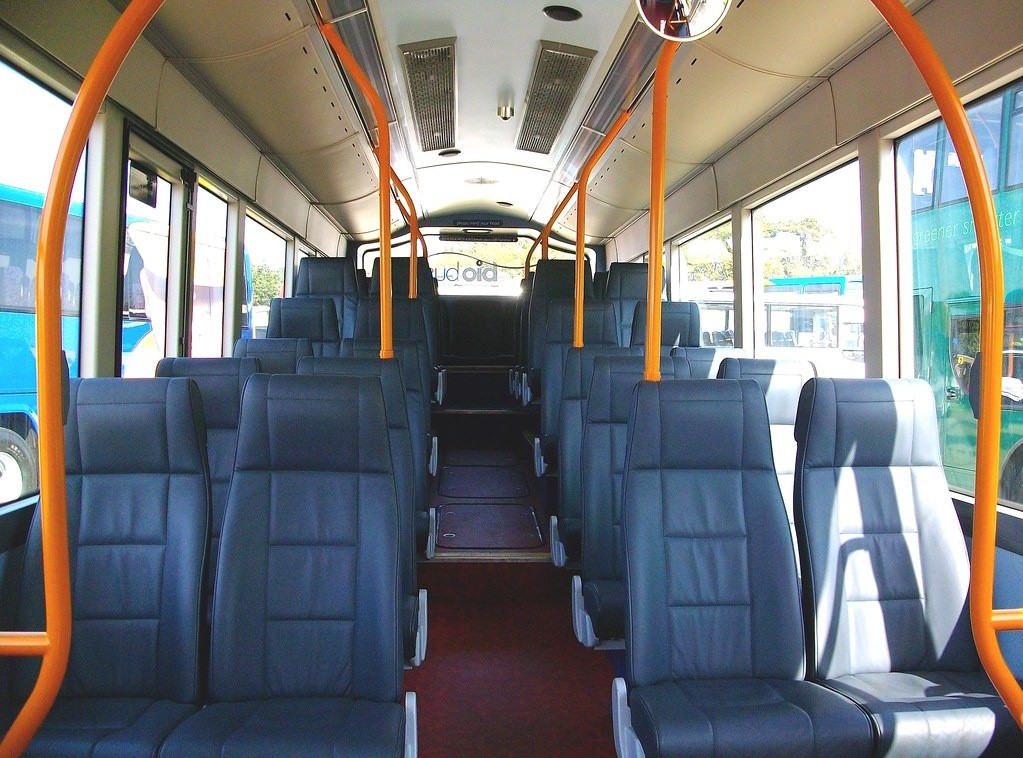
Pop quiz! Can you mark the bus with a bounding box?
[895,79,1022,514]
[0,182,254,505]
[682,293,865,426]
[683,274,862,334]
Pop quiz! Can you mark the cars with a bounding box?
[242,305,270,328]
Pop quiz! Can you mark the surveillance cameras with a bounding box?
[497,106,514,120]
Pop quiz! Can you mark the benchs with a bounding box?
[0,255,445,758]
[443,259,1023,757]
